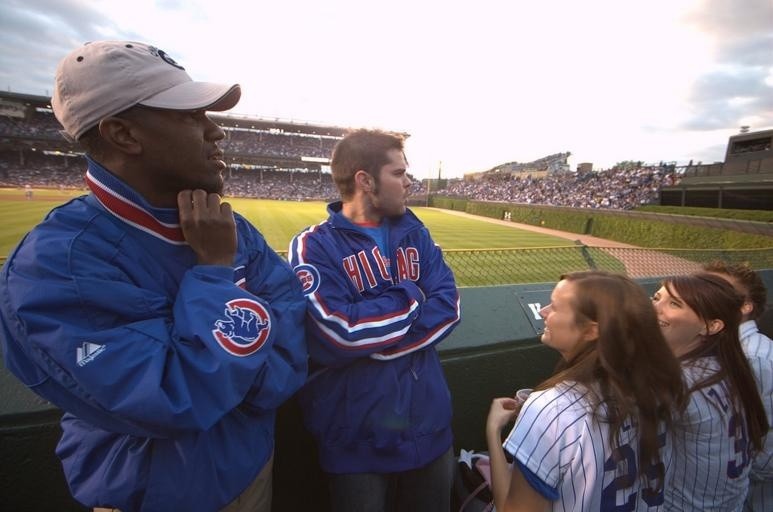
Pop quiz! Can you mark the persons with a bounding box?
[464,266,694,509]
[1,37,311,509]
[404,164,682,212]
[285,125,464,509]
[216,130,343,200]
[632,272,773,511]
[1,110,88,199]
[697,255,773,509]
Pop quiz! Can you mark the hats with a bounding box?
[49,40,241,144]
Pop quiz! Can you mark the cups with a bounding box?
[514,388,535,417]
